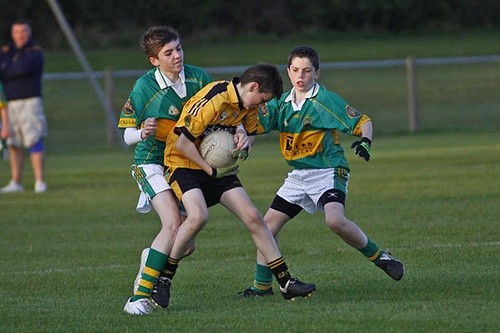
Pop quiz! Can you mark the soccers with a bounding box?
[199,131,238,168]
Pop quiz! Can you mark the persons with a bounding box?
[150,65,317,309]
[243,47,404,296]
[118,28,216,315]
[0,22,47,194]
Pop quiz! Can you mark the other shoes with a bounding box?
[34,182,46,193]
[0,183,24,192]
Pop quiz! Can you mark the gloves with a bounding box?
[231,148,251,161]
[350,137,372,162]
[211,165,239,178]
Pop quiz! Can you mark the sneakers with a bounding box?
[280,278,315,303]
[238,288,274,296]
[124,298,155,315]
[134,248,150,296]
[151,277,171,309]
[378,251,404,281]
